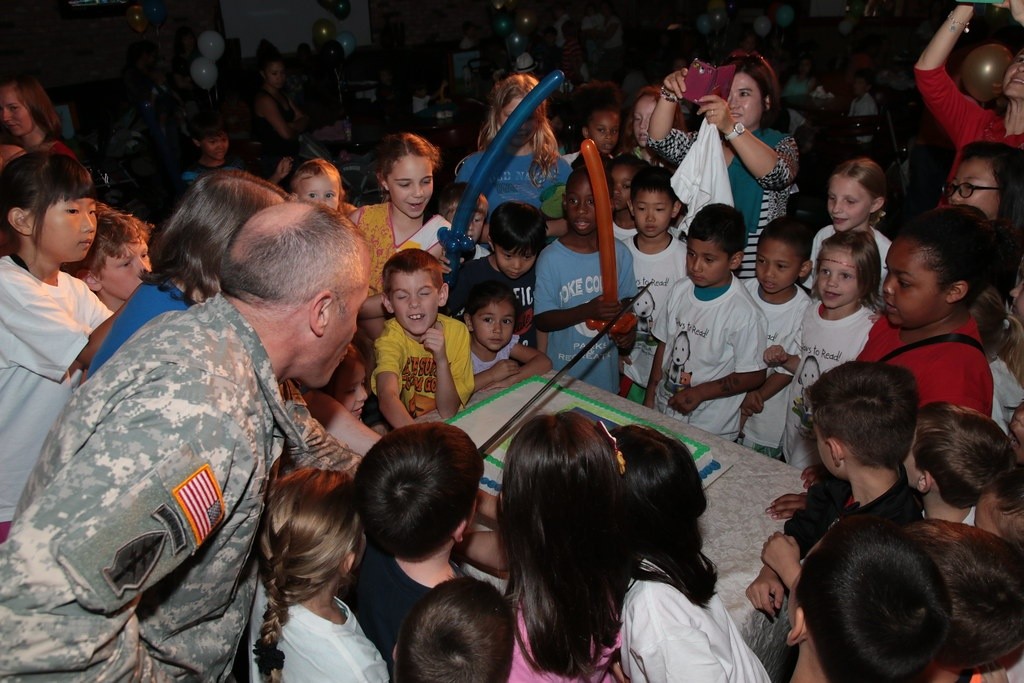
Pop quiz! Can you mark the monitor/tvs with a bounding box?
[58,0,137,21]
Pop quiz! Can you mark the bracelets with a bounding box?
[725,122,745,141]
[660,84,677,102]
[948,10,970,33]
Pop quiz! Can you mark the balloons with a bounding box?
[984,3,1009,29]
[726,1,737,15]
[697,14,711,34]
[197,30,225,61]
[768,1,784,24]
[190,57,218,90]
[320,0,336,10]
[336,30,356,56]
[127,4,148,32]
[505,0,518,9]
[319,40,344,68]
[707,0,726,15]
[508,32,529,56]
[331,0,350,20]
[438,69,565,283]
[581,139,636,336]
[515,7,538,35]
[711,8,728,31]
[313,18,336,46]
[775,5,795,28]
[961,43,1014,102]
[144,0,167,26]
[492,13,514,39]
[754,16,771,38]
[492,0,504,10]
[852,0,864,16]
[838,21,853,35]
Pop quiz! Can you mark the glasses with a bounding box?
[723,52,765,68]
[943,182,999,198]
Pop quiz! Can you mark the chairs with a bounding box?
[812,112,918,161]
[406,123,476,166]
[230,139,262,177]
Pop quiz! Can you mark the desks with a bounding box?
[356,367,812,683]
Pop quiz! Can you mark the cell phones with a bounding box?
[682,59,716,106]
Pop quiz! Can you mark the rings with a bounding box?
[711,110,713,115]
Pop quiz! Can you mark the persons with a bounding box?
[0,0,1024,683]
